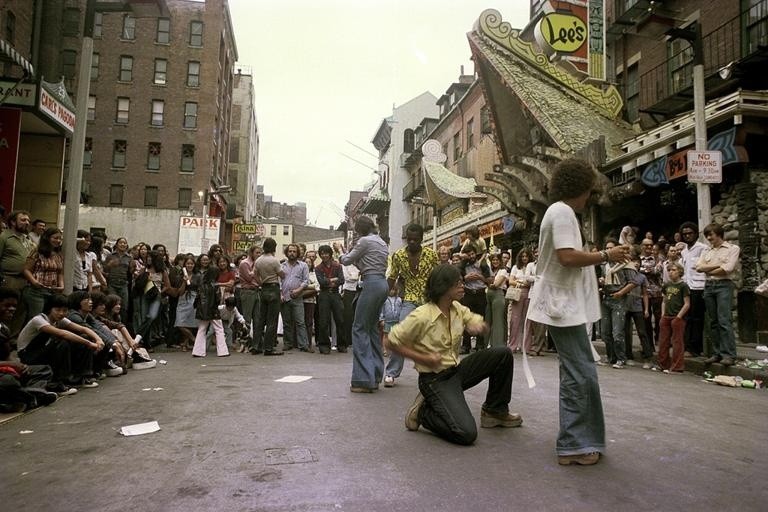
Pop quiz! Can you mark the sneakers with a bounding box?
[301,347,348,353]
[252,350,262,355]
[459,347,469,353]
[704,355,718,363]
[597,357,682,375]
[264,349,283,355]
[282,347,291,351]
[720,357,735,364]
[39,360,127,406]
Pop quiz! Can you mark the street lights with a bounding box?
[201,185,234,256]
[409,195,437,252]
[623,13,712,249]
[62,0,171,298]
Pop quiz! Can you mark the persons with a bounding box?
[520,157,633,465]
[382,264,525,444]
[2,211,740,414]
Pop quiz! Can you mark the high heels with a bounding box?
[559,453,599,465]
[384,375,394,386]
[133,354,156,371]
[350,385,377,394]
[480,408,522,429]
[405,390,425,431]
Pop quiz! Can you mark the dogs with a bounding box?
[237,327,253,354]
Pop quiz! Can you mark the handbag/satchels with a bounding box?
[504,287,522,301]
